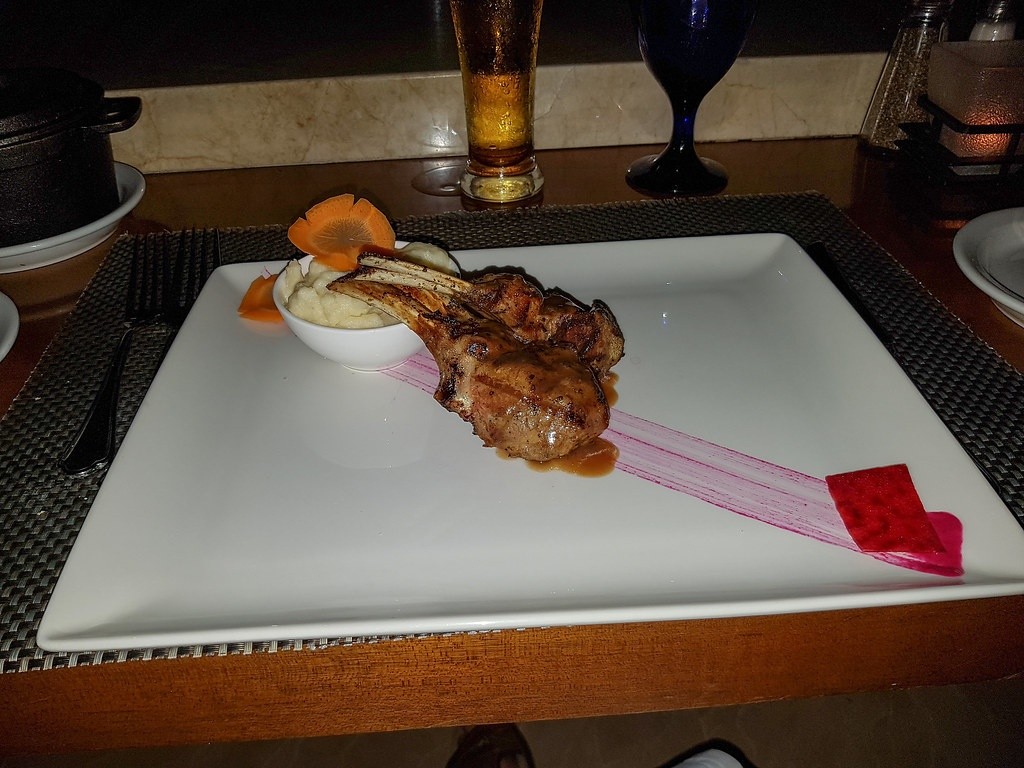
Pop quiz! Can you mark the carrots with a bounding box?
[288,193,396,273]
[238,274,287,322]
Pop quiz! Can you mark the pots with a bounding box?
[0,82,143,247]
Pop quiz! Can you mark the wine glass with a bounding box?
[625,0,758,196]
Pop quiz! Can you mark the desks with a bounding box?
[0,129,1024,768]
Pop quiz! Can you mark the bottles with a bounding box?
[969,1,1019,43]
[859,0,953,150]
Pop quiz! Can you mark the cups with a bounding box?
[927,41,1024,229]
[448,1,545,203]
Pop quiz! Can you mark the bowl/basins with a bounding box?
[272,241,461,371]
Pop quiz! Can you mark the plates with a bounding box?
[0,290,21,360]
[954,206,1024,328]
[1,160,145,273]
[34,232,1024,654]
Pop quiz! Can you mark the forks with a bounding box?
[58,234,171,477]
[154,227,222,374]
